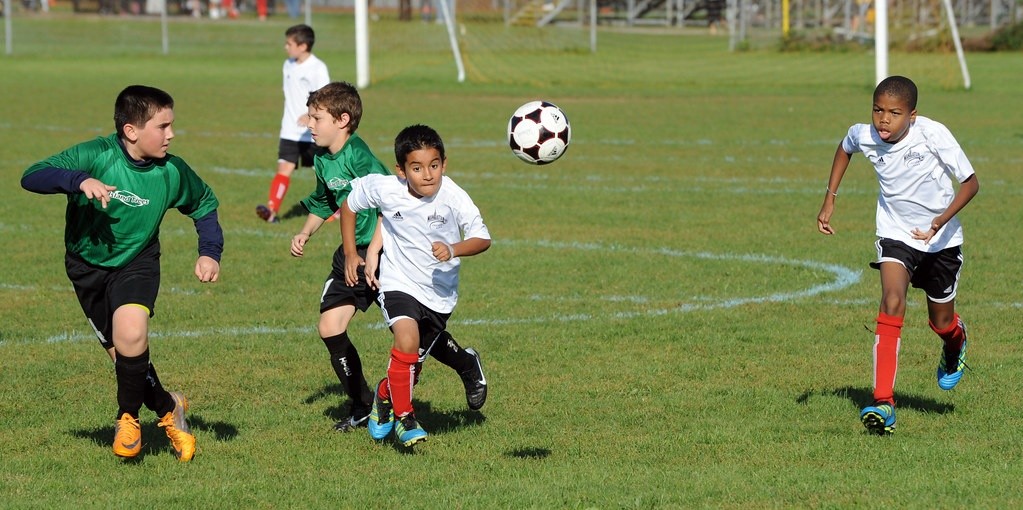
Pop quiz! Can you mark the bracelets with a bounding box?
[826,187,839,196]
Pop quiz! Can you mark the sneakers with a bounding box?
[368,377,394,438]
[393,411,427,447]
[157,391,195,461]
[255,205,279,222]
[938,320,967,390]
[458,347,487,410]
[112,412,142,456]
[333,411,372,433]
[860,404,896,435]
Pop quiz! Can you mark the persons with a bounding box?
[340,123,492,447]
[699,0,728,33]
[289,82,489,431]
[96,0,268,22]
[817,77,979,436]
[256,25,339,224]
[20,85,224,463]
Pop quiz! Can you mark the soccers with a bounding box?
[506,100,572,166]
